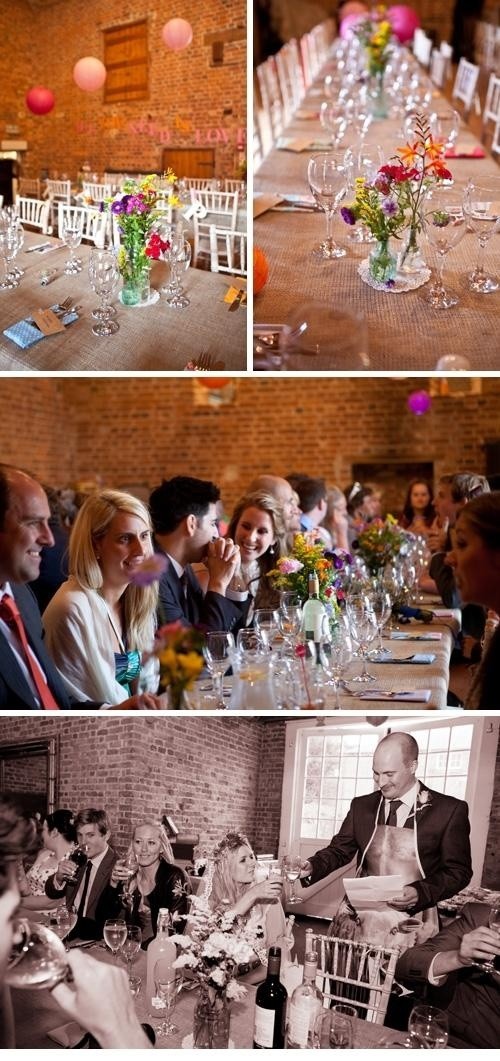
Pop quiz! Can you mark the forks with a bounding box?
[193,351,214,371]
[53,294,73,315]
[253,322,309,350]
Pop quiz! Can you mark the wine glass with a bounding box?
[118,853,139,900]
[283,855,305,906]
[316,31,463,190]
[160,232,192,308]
[90,244,122,338]
[1,205,26,293]
[306,153,351,261]
[200,532,430,708]
[61,212,85,276]
[343,142,385,244]
[63,842,91,881]
[2,905,143,1009]
[152,957,184,1037]
[458,174,500,292]
[419,191,466,310]
[472,897,499,975]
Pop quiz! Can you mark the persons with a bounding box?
[0,803,160,1050]
[179,831,296,991]
[46,807,123,939]
[93,819,192,953]
[290,732,475,1031]
[15,810,79,917]
[0,467,499,711]
[393,903,499,1049]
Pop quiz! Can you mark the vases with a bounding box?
[161,691,188,711]
[366,241,397,287]
[399,228,427,273]
[117,255,160,305]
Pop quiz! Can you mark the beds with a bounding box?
[0,223,249,370]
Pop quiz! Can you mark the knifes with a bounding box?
[26,244,52,254]
[59,306,82,319]
[228,289,244,313]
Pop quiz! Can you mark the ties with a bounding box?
[181,574,190,601]
[77,861,92,919]
[1,592,60,710]
[386,800,403,826]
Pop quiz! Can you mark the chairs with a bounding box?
[414,22,500,169]
[187,876,203,924]
[250,8,342,183]
[0,162,247,277]
[302,929,401,1030]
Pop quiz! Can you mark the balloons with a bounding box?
[407,390,432,415]
[25,86,57,116]
[73,56,109,93]
[337,1,420,44]
[162,17,194,52]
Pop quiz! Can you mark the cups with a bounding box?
[330,1004,358,1048]
[409,1005,451,1048]
[312,1011,353,1047]
[264,871,282,904]
[279,301,370,370]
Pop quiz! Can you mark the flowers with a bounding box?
[383,111,452,270]
[271,523,338,609]
[336,3,430,59]
[144,619,203,709]
[339,169,407,280]
[352,511,410,567]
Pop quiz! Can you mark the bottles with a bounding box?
[395,226,427,277]
[146,907,176,1019]
[287,949,323,1048]
[253,945,287,1048]
[367,243,399,285]
